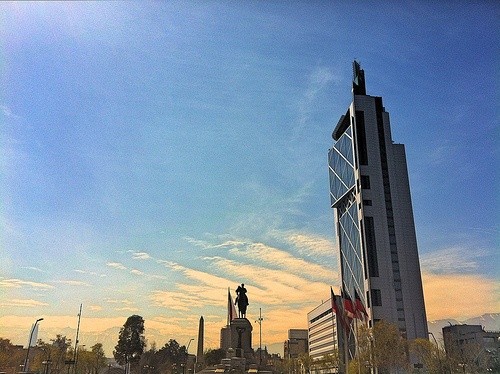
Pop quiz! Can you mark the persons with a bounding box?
[234,284,250,306]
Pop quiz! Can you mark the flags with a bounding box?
[342,282,363,322]
[330,288,347,333]
[339,288,353,341]
[352,287,369,324]
